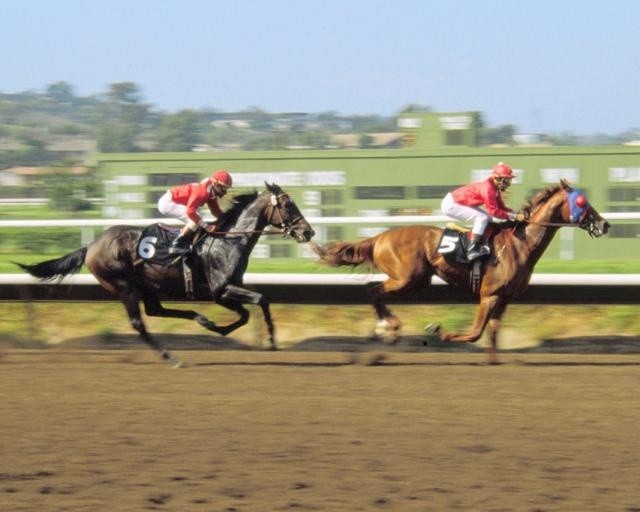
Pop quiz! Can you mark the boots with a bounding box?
[168,227,195,254]
[464,233,492,261]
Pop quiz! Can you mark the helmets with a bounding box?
[491,161,517,180]
[211,170,233,188]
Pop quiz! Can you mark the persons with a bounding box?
[439,161,527,262]
[155,170,233,254]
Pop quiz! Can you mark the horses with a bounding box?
[9,179,316,369]
[308,177,612,365]
